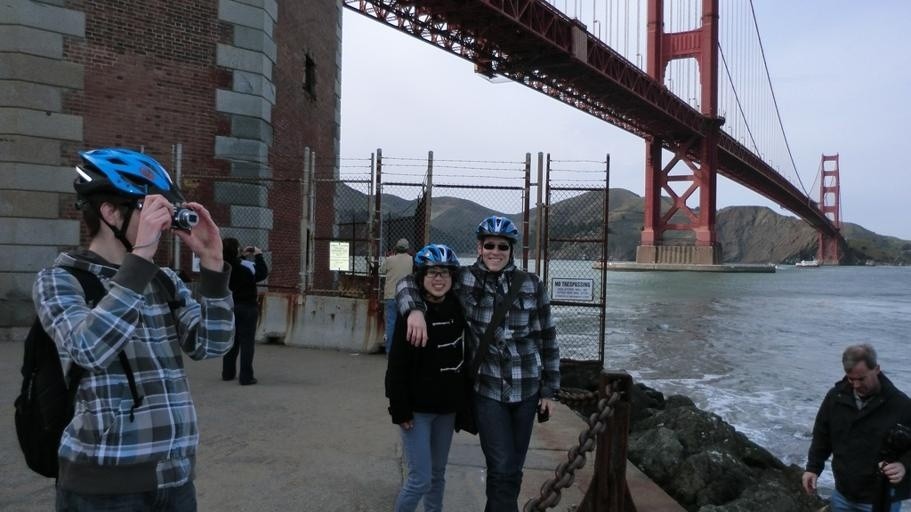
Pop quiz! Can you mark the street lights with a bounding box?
[668,79,675,92]
[693,98,698,109]
[727,125,793,183]
[593,20,601,41]
[636,53,643,70]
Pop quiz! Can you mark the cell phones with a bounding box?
[538,405,549,423]
[247,247,255,252]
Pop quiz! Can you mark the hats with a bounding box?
[396,238,410,251]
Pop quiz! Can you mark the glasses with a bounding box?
[425,271,453,278]
[484,243,511,251]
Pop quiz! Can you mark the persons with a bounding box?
[396,216,563,512]
[220,238,271,385]
[803,342,910,512]
[387,242,470,512]
[32,144,238,511]
[381,238,415,354]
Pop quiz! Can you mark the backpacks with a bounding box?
[13,264,177,480]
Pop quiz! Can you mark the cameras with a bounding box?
[170,201,199,231]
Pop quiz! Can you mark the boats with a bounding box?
[796,260,819,268]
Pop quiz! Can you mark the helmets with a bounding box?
[475,216,520,244]
[72,147,188,211]
[416,243,461,270]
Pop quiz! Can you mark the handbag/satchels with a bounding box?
[454,389,480,436]
